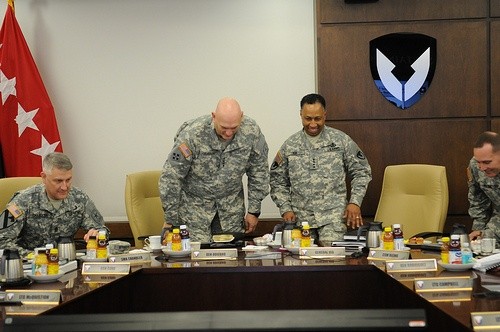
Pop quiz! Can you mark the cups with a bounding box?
[275,232,281,243]
[481,229,497,255]
[144,236,161,249]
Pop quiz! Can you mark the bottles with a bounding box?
[86,232,107,258]
[292,221,311,247]
[31,244,60,275]
[165,225,189,250]
[382,224,404,249]
[439,234,473,264]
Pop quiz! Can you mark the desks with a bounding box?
[0,247,500,332]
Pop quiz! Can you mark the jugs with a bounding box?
[0,247,24,282]
[273,221,296,245]
[366,221,384,247]
[53,233,76,261]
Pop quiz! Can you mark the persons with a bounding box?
[158,98,271,234]
[270,94,372,243]
[465,132,500,251]
[0,152,111,261]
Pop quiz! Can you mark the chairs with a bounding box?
[375,163,450,242]
[0,177,43,213]
[125,169,164,248]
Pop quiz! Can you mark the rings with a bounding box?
[356,217,359,219]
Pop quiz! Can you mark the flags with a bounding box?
[0,5,67,177]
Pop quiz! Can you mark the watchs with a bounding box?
[248,212,260,217]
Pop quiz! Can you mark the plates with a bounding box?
[27,271,64,283]
[435,260,476,273]
[252,237,318,253]
[376,246,412,251]
[404,241,423,246]
[79,245,191,258]
